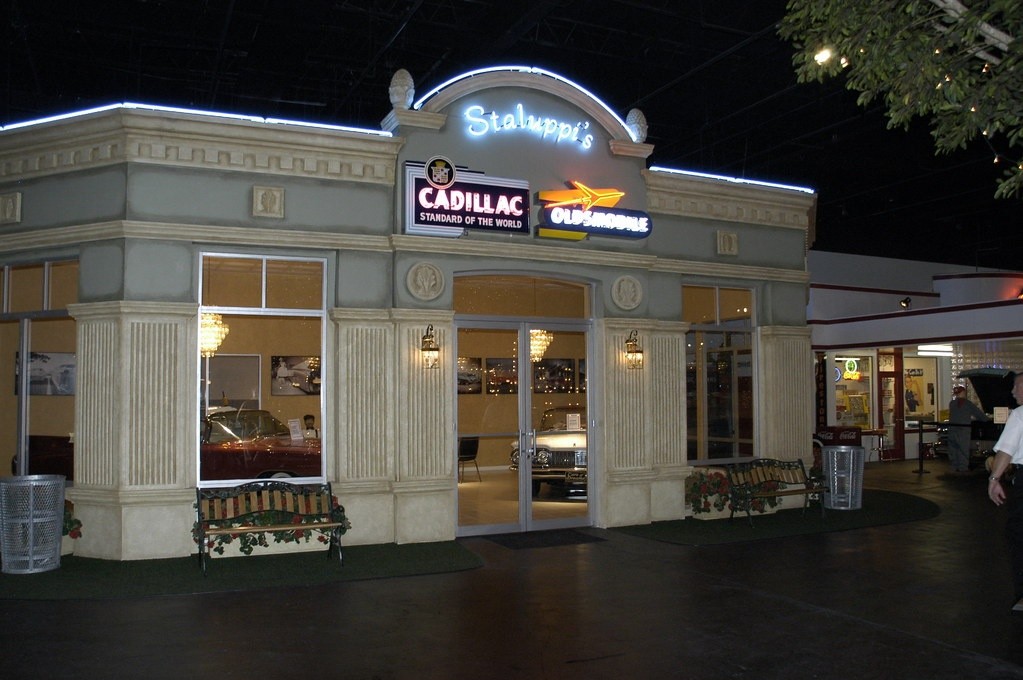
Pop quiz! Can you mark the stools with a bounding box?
[868,429,893,465]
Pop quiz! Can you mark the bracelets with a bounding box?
[988,476,1000,481]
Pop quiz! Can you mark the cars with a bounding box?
[509,403,588,498]
[199,403,322,482]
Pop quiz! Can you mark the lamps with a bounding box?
[530,279,553,362]
[900,297,912,310]
[626,329,644,369]
[201,256,230,358]
[421,323,440,370]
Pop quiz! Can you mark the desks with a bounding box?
[905,415,934,474]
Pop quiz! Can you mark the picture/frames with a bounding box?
[457,357,483,394]
[534,359,576,393]
[486,358,518,394]
[270,355,320,396]
[14,351,75,396]
[578,359,586,393]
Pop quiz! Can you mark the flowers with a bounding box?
[686,469,787,515]
[62,511,83,539]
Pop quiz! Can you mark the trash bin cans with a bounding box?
[1,473,69,573]
[821,445,865,511]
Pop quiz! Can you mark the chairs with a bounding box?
[458,437,482,484]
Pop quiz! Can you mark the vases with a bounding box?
[692,485,809,522]
[60,534,73,556]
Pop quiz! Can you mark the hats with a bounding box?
[952,386,964,396]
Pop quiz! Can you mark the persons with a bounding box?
[986,373,1023,613]
[905,388,920,412]
[275,360,289,385]
[303,415,321,439]
[948,387,990,473]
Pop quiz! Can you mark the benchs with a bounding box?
[192,480,352,574]
[725,458,829,530]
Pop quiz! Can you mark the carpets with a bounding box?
[608,487,941,546]
[0,541,486,602]
[482,529,609,551]
